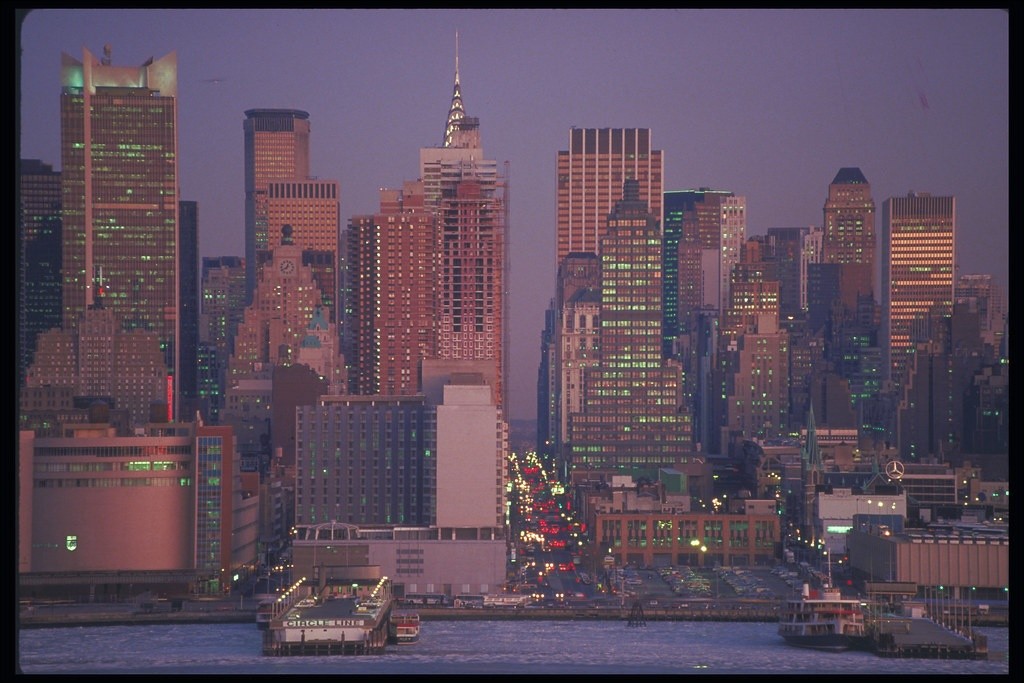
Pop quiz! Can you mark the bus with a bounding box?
[484,594,531,608]
[404,594,448,605]
[455,595,484,606]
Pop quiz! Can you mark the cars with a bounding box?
[615,565,643,594]
[658,565,711,597]
[714,566,769,597]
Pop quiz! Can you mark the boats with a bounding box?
[255,600,282,630]
[777,548,866,652]
[389,610,421,645]
[773,566,809,591]
